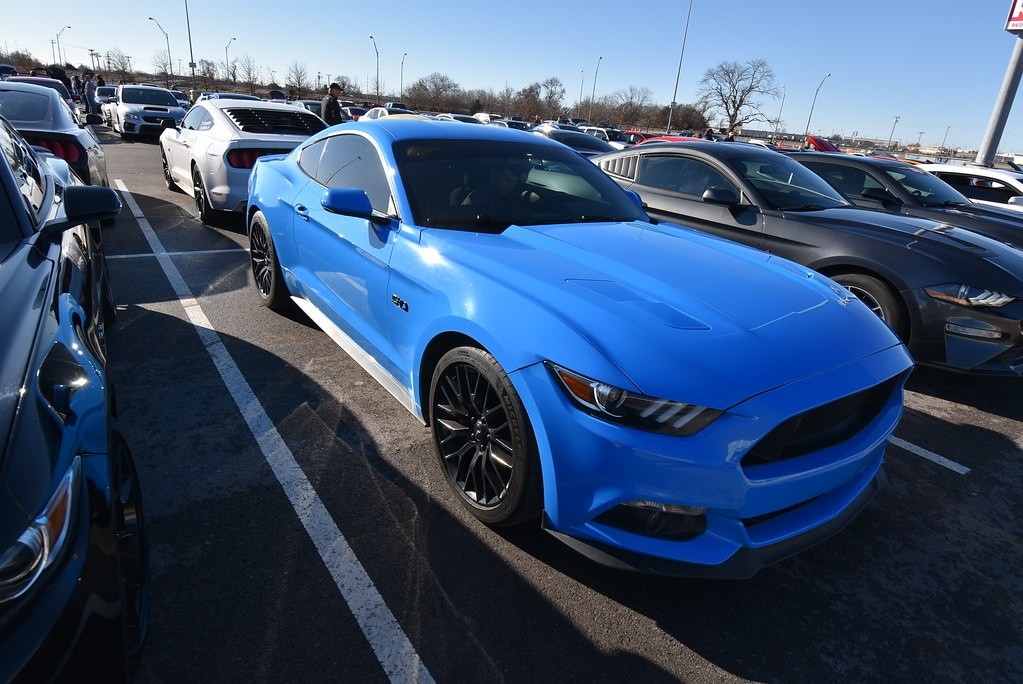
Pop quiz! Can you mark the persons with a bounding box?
[10,70,17,76]
[723,132,734,142]
[171,85,187,100]
[768,140,778,146]
[70,74,97,116]
[96,74,105,86]
[29,70,36,76]
[461,158,519,208]
[321,83,344,127]
[796,141,818,150]
[692,128,714,141]
[557,116,564,124]
[534,116,541,126]
[118,80,136,85]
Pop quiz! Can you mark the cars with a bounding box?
[194,92,528,131]
[0,114,124,684]
[0,64,81,122]
[94,83,192,141]
[748,137,1023,213]
[0,81,115,229]
[525,141,1023,378]
[245,118,915,567]
[158,99,331,225]
[743,152,1023,251]
[527,119,725,158]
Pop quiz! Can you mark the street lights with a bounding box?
[56,26,71,66]
[225,38,236,93]
[578,70,583,119]
[589,57,602,122]
[802,73,831,141]
[149,18,174,86]
[772,85,785,142]
[370,36,379,104]
[400,53,407,104]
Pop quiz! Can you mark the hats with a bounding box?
[707,129,715,134]
[329,83,344,91]
